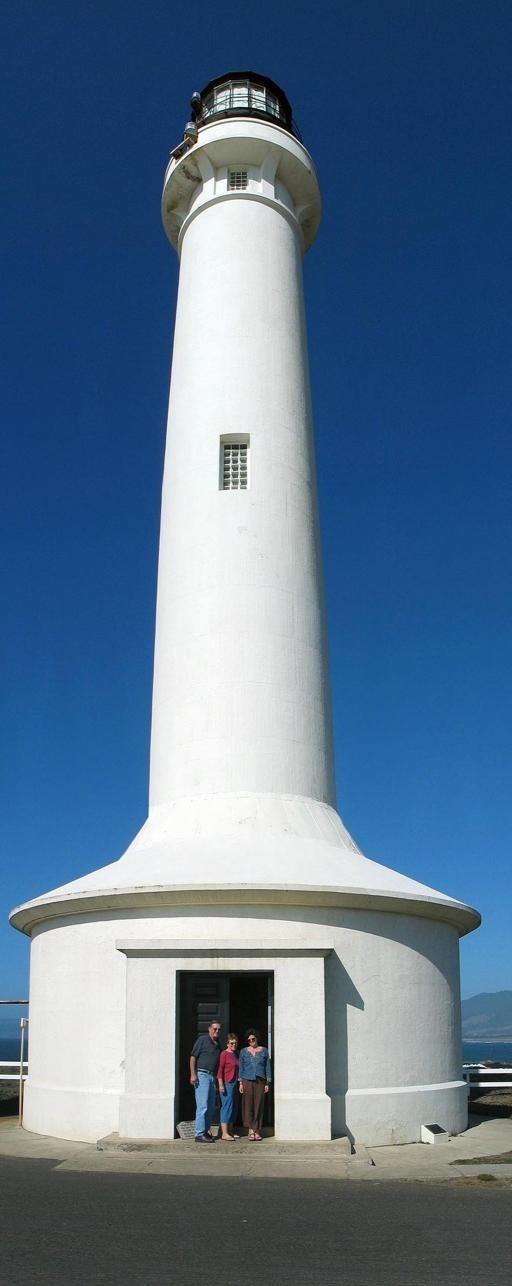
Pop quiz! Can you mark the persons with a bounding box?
[189,1019,222,1144]
[215,1031,241,1141]
[236,1026,272,1141]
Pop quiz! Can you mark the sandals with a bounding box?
[247,1131,262,1141]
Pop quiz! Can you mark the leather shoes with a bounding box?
[195,1133,216,1143]
[221,1131,240,1141]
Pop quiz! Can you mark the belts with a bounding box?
[197,1069,215,1076]
